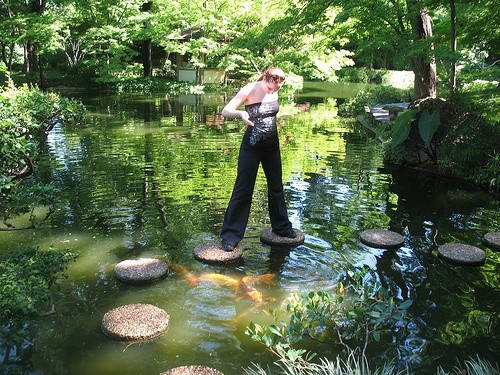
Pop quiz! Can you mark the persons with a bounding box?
[219,66,299,250]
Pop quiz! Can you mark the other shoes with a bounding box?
[277,230,297,239]
[222,243,235,251]
